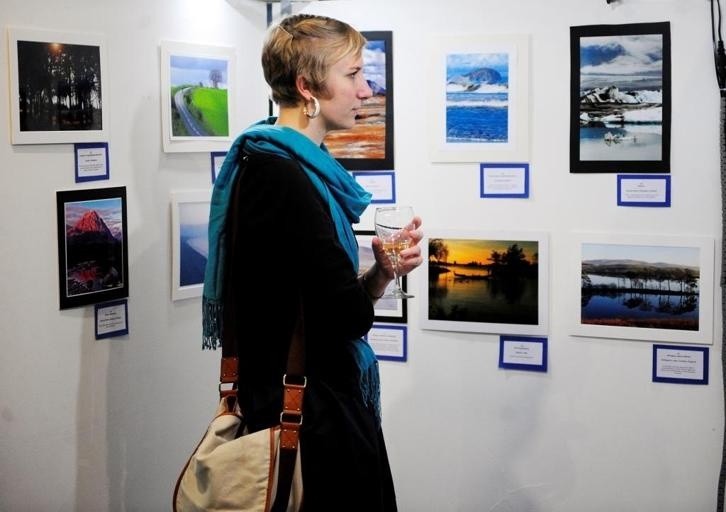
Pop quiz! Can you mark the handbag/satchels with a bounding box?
[174,390,304,512]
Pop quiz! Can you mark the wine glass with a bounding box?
[374,204,417,300]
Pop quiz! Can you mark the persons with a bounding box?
[202,15,422,511]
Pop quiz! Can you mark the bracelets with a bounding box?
[362,273,385,300]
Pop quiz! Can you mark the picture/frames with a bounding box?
[56,185,129,313]
[8,27,110,143]
[160,38,237,154]
[168,189,221,308]
[321,28,393,168]
[435,33,530,161]
[350,229,407,321]
[568,231,714,344]
[419,231,550,339]
[569,24,671,173]
